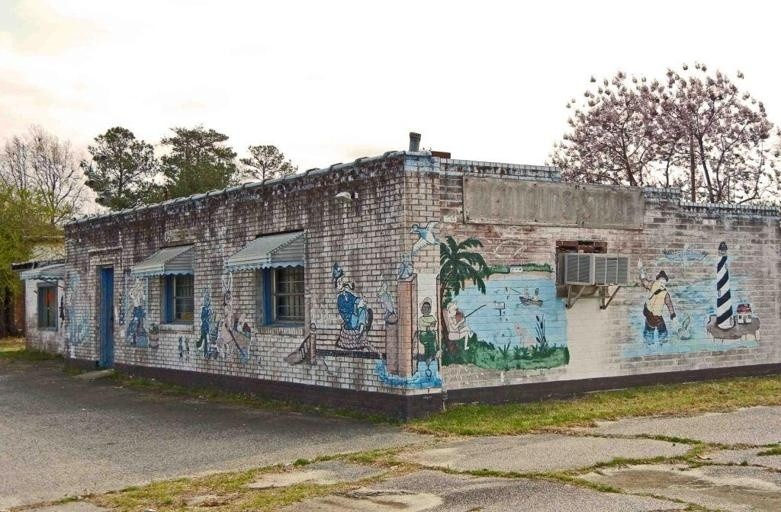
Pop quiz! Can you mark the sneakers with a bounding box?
[358,330,367,344]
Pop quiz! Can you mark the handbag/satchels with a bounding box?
[643,303,649,316]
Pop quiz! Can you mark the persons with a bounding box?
[637,257,677,342]
[127,278,143,343]
[197,288,233,360]
[332,262,369,341]
[377,284,396,318]
[419,297,437,361]
[523,288,539,301]
[447,302,473,350]
[178,340,189,362]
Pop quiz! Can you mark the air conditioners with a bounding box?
[563,252,629,286]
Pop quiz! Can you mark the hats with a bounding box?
[656,271,668,282]
[332,262,344,288]
